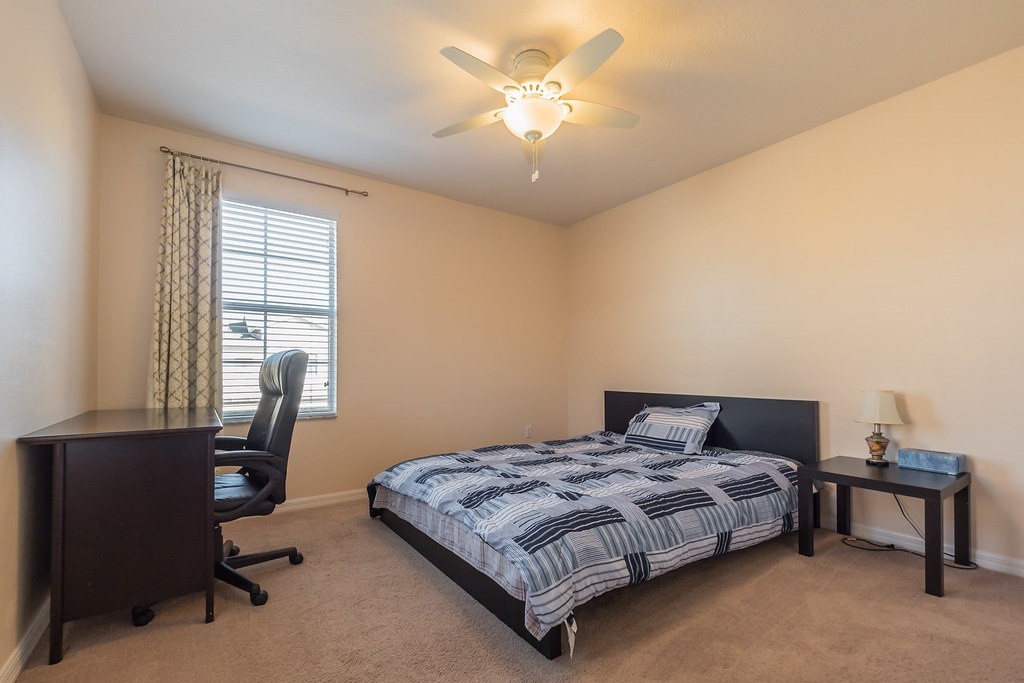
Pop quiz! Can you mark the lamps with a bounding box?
[500,97,566,183]
[854,388,905,468]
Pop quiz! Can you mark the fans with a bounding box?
[430,27,642,139]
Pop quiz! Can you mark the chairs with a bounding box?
[209,349,312,612]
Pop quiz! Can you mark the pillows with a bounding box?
[622,401,721,458]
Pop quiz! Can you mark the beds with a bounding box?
[365,389,820,661]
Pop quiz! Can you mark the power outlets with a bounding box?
[524,424,535,442]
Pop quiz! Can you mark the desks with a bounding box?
[796,456,972,598]
[15,405,223,666]
